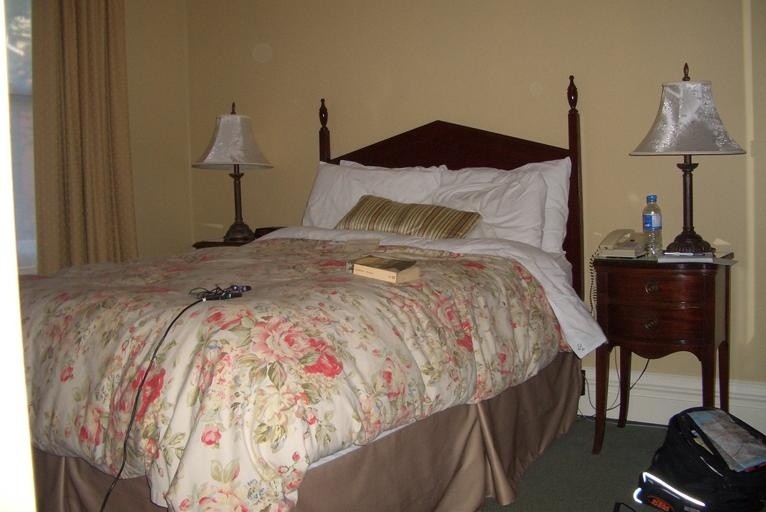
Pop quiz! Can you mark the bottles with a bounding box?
[642,195,663,255]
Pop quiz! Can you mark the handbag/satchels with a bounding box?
[631,402,765,510]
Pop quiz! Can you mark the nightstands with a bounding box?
[587,255,737,455]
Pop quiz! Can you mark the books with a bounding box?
[659,247,713,265]
[344,253,425,286]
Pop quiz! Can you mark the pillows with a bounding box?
[302,154,572,251]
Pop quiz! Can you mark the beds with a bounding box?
[19,74,590,512]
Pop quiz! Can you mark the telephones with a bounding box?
[597,229,647,258]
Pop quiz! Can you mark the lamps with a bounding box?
[187,100,276,245]
[629,60,748,255]
